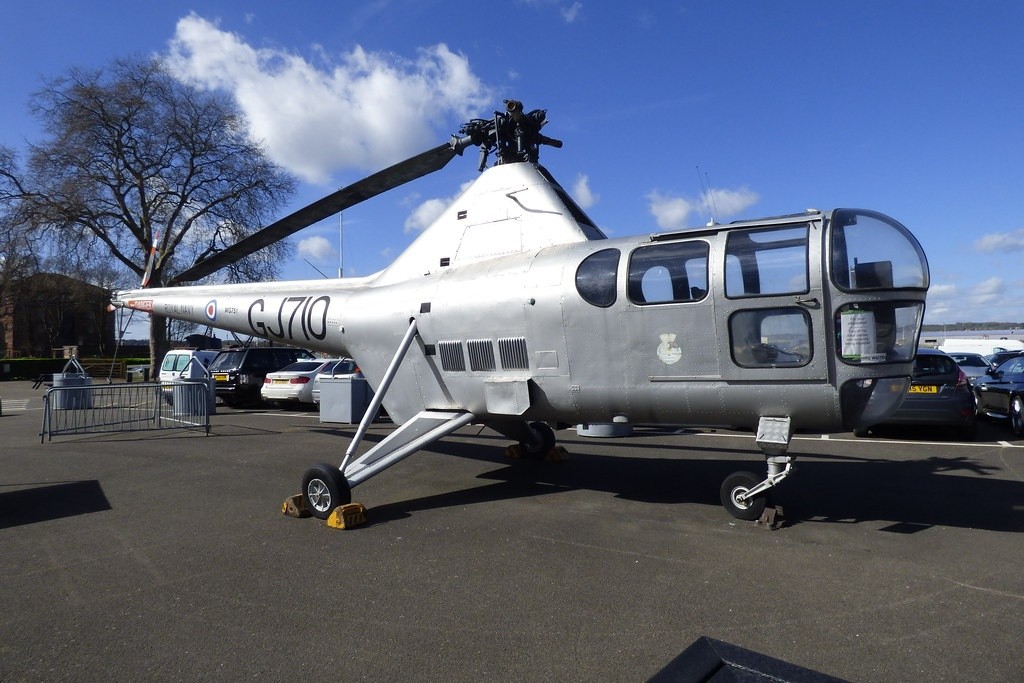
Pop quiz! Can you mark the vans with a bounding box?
[158,346,218,405]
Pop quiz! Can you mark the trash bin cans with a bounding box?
[52,355,94,411]
[171,356,218,416]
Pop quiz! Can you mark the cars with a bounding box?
[259,358,350,410]
[853,347,979,440]
[945,352,996,389]
[972,355,1024,436]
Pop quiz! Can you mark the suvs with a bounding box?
[204,347,322,409]
[989,350,1024,372]
[312,359,366,412]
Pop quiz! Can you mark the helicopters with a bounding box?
[106,96,934,516]
